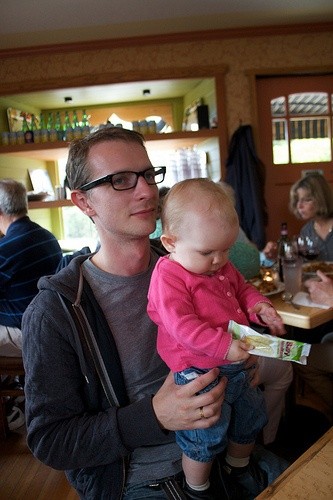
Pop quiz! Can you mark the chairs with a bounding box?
[0,247,91,439]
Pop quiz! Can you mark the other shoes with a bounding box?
[221,459,268,495]
[181,482,223,500]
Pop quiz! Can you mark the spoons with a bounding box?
[281,292,299,310]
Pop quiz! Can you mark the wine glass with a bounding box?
[305,234,321,274]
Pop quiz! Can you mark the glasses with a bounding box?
[78,166,166,191]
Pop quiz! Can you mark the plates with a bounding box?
[245,279,285,296]
[301,261,333,276]
[26,194,52,200]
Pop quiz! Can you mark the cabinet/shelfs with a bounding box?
[0,65,231,210]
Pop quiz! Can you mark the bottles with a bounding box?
[275,230,289,283]
[2,131,25,146]
[196,98,210,130]
[133,120,156,136]
[22,109,90,143]
[265,246,280,281]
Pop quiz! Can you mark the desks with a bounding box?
[250,265,333,341]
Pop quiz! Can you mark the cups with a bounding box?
[281,242,299,265]
[258,269,274,282]
[55,188,67,200]
[291,234,305,258]
[279,255,303,296]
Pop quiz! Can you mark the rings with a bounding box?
[199,406,205,420]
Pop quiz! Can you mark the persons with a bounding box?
[289,169,333,261]
[22,128,293,500]
[145,177,287,500]
[0,180,63,357]
[304,270,333,344]
[149,183,260,278]
